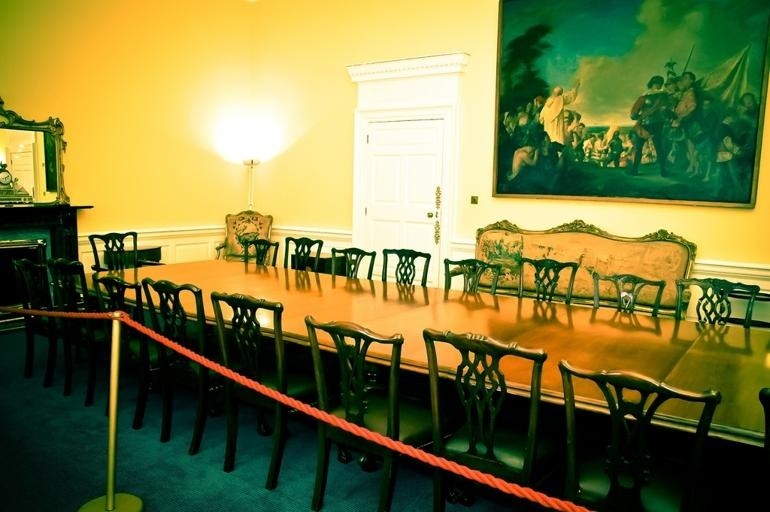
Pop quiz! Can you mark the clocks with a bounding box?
[0,161,12,184]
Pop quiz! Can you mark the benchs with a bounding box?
[475,216,701,321]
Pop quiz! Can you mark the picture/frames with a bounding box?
[492,0,769,209]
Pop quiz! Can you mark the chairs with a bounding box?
[13,255,63,392]
[442,255,505,294]
[207,291,316,493]
[243,237,279,269]
[304,314,458,510]
[213,211,277,265]
[673,272,761,331]
[420,323,559,511]
[282,236,324,272]
[586,268,668,317]
[329,246,379,281]
[517,256,579,304]
[49,258,115,409]
[556,357,724,512]
[380,247,432,286]
[92,271,175,434]
[89,231,141,276]
[142,278,224,457]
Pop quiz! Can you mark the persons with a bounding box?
[500,71,759,204]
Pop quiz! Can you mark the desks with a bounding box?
[85,258,769,469]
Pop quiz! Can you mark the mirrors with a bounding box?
[0,98,70,208]
